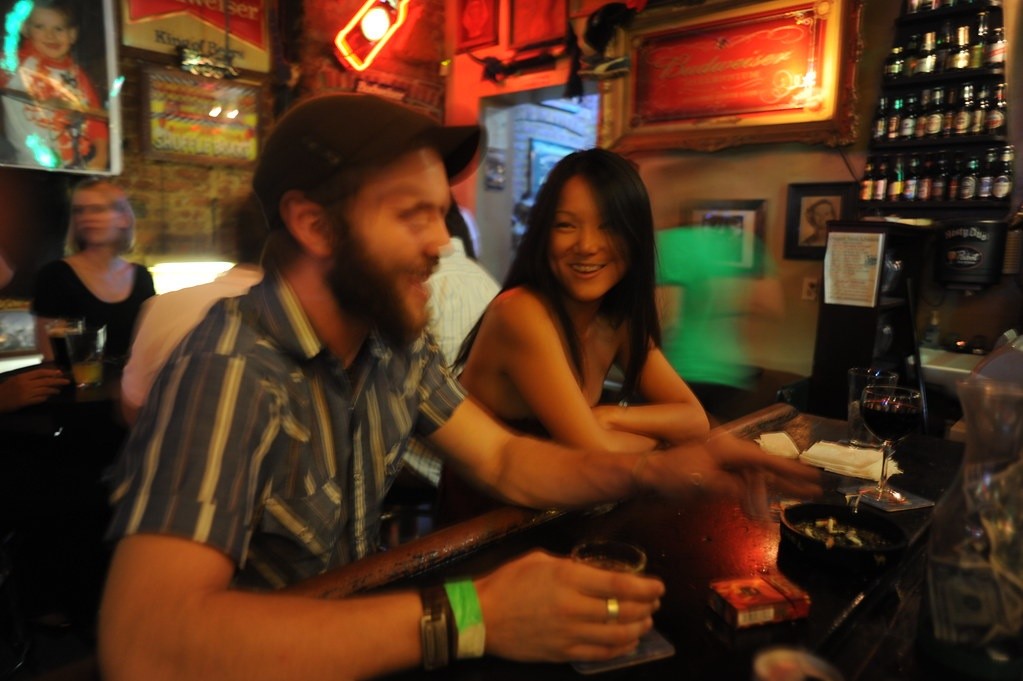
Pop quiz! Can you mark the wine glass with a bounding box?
[855,371,928,504]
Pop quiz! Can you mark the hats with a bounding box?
[252,92,488,230]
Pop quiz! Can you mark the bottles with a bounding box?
[860,1,1015,202]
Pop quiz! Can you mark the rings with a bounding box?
[605,597,620,627]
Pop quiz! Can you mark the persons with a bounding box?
[99,92,674,678]
[0,0,109,169]
[452,147,712,458]
[0,367,73,410]
[800,200,839,246]
[120,197,279,434]
[28,178,164,380]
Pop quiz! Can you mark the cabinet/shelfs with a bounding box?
[858,0,1023,214]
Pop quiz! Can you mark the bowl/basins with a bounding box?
[775,497,907,602]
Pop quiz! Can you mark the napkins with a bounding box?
[755,430,906,481]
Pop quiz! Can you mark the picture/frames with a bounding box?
[138,61,276,169]
[783,181,858,261]
[678,197,767,279]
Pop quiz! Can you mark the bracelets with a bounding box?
[417,582,459,679]
[446,577,486,661]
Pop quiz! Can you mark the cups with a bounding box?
[847,366,899,448]
[569,538,647,575]
[64,321,107,390]
[46,319,86,378]
